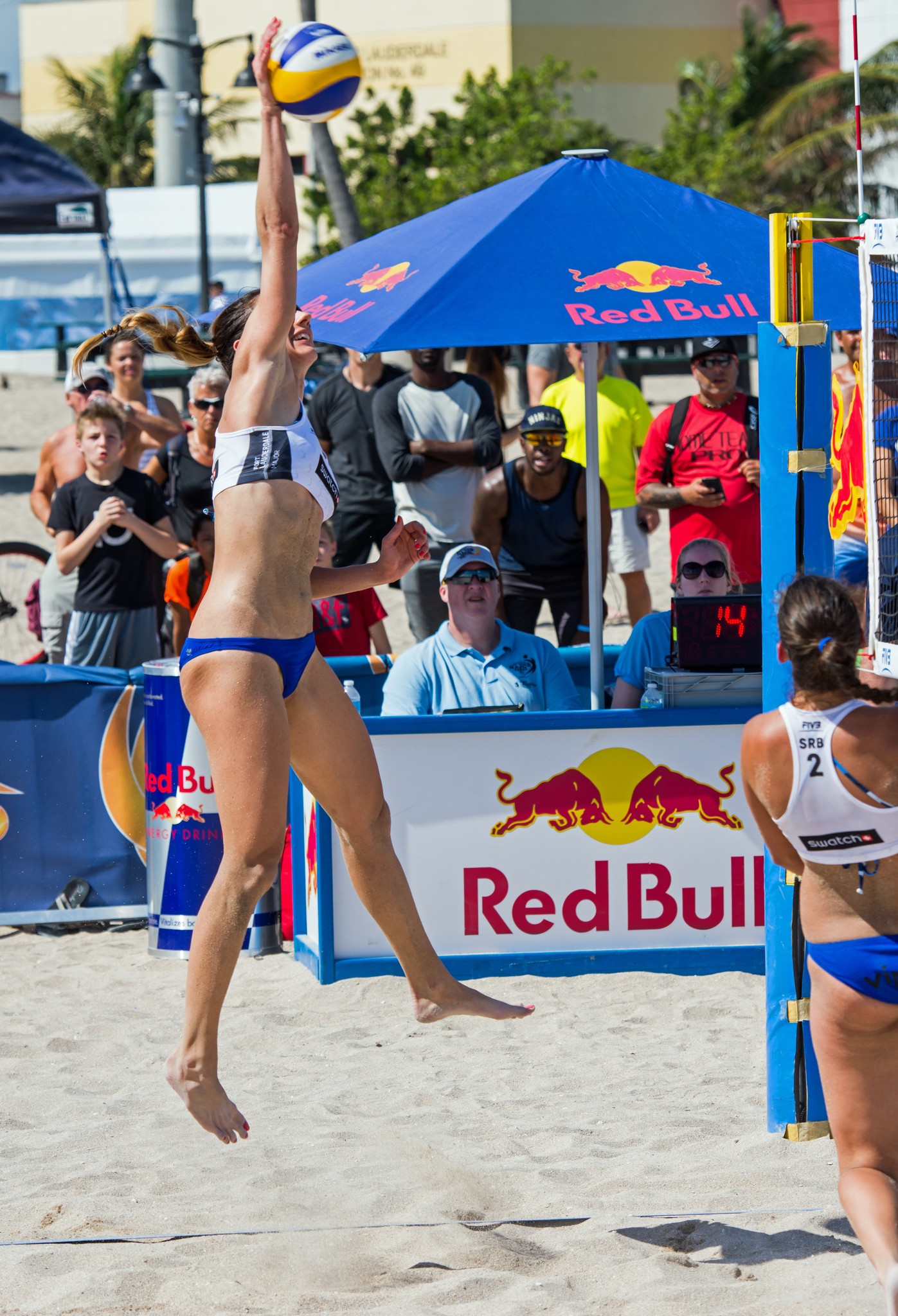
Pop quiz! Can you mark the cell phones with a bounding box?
[702,478,726,500]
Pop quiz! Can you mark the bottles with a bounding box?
[640,683,664,708]
[343,680,361,718]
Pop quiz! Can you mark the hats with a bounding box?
[691,335,738,362]
[519,406,568,435]
[65,361,109,394]
[439,543,500,586]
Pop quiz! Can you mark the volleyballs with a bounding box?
[264,20,361,124]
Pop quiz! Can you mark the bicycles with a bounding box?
[0,542,51,668]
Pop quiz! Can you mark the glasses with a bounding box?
[521,433,567,447]
[189,397,224,410]
[76,384,108,395]
[677,560,729,581]
[573,341,603,351]
[443,567,497,585]
[691,353,738,370]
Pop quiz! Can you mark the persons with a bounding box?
[635,336,762,596]
[381,544,580,715]
[739,568,898,1316]
[830,329,898,649]
[165,17,536,1147]
[31,278,659,714]
[611,538,744,710]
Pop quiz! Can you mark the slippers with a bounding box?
[33,879,147,936]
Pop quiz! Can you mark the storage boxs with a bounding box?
[645,666,764,707]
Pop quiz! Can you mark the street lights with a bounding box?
[126,33,256,335]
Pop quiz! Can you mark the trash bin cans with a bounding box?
[142,658,278,956]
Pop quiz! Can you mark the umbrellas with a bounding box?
[183,147,898,709]
[1,116,108,213]
